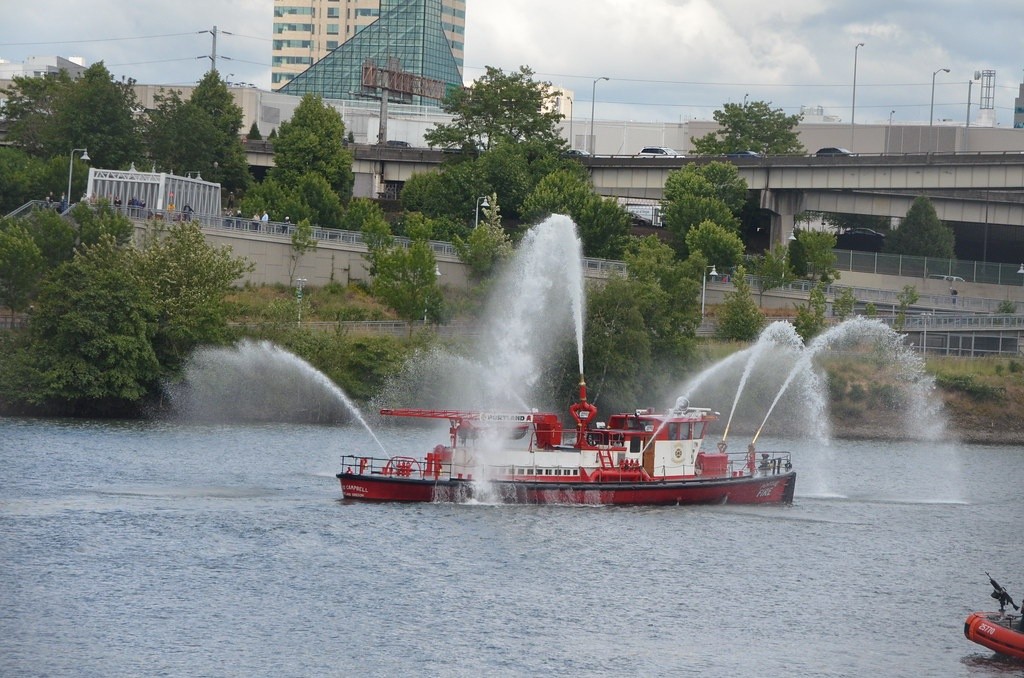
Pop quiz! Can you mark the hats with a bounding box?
[285,216,289,220]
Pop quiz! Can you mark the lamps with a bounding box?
[597,422,606,428]
[645,425,655,431]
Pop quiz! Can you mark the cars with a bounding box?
[815,148,859,156]
[729,150,761,158]
[845,228,883,238]
[626,213,652,225]
[563,150,591,157]
[386,140,413,147]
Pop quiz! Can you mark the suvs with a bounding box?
[638,146,685,158]
[929,275,964,281]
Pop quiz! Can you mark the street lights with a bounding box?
[852,43,864,151]
[591,77,610,155]
[928,69,951,156]
[701,265,718,321]
[475,195,490,228]
[567,96,572,148]
[423,264,442,323]
[296,277,307,327]
[68,149,90,207]
[887,110,896,153]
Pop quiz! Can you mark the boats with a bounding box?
[334,372,796,506]
[963,570,1023,660]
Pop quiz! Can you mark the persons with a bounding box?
[43,192,291,235]
[949,280,959,307]
